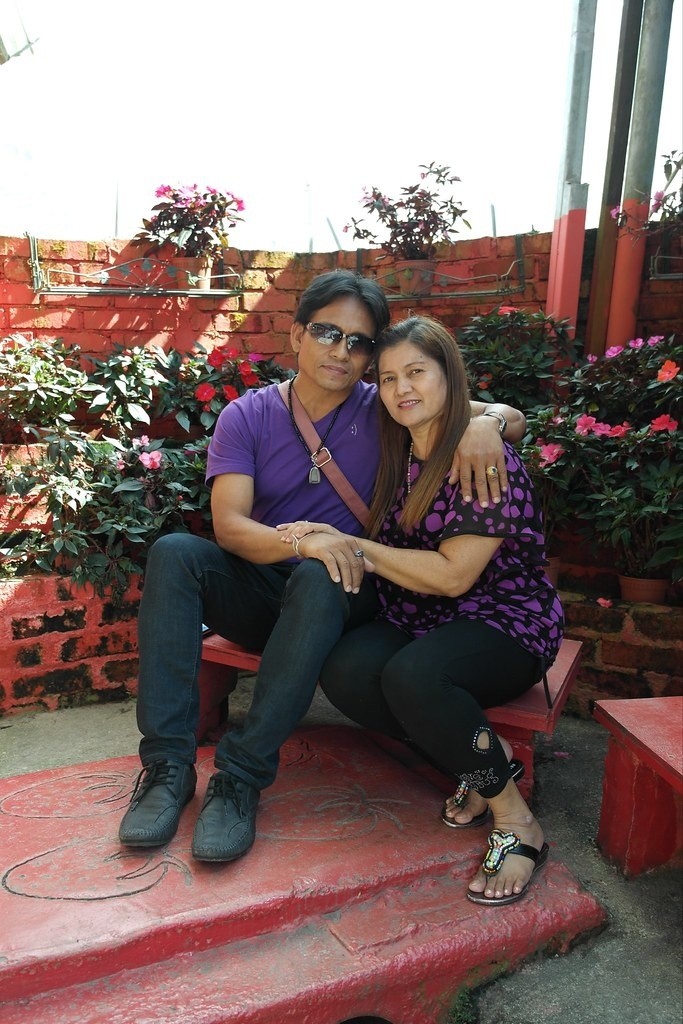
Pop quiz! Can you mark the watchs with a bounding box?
[483,412,507,434]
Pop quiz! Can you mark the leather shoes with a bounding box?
[191,770,260,861]
[119,761,197,845]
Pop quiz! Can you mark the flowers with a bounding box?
[511,405,582,557]
[555,334,683,423]
[130,181,246,268]
[90,434,212,561]
[517,403,683,578]
[342,161,473,261]
[610,151,683,258]
[155,346,295,434]
[453,300,583,404]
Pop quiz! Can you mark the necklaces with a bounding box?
[287,375,343,484]
[408,442,414,493]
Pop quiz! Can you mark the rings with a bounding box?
[486,466,498,475]
[306,521,308,523]
[309,522,310,524]
[355,550,364,557]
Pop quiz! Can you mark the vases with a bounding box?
[395,260,440,295]
[543,557,562,589]
[177,420,211,441]
[616,571,669,603]
[172,257,214,289]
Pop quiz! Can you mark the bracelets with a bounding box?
[291,533,317,559]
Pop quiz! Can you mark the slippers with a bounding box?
[441,758,525,828]
[467,829,550,906]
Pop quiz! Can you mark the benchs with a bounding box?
[199,635,583,802]
[592,697,683,881]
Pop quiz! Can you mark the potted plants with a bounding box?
[78,341,182,439]
[0,333,93,442]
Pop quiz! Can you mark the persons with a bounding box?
[117,272,564,904]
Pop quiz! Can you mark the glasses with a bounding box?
[306,320,376,357]
